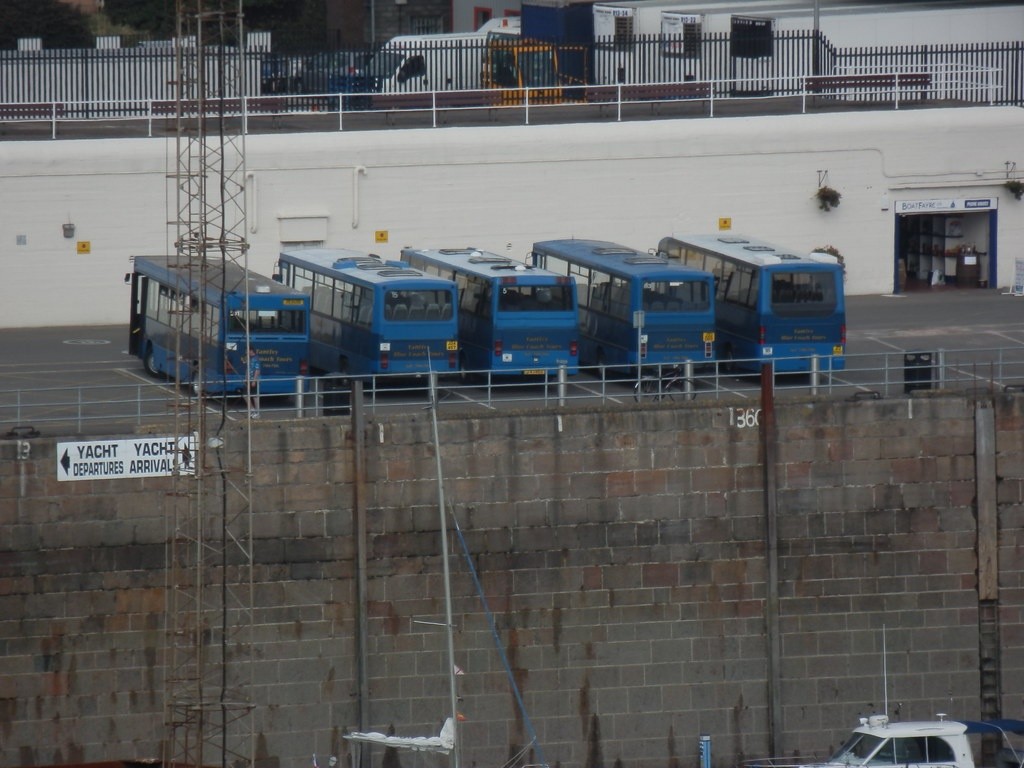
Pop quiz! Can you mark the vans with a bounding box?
[295,49,370,93]
[362,33,485,93]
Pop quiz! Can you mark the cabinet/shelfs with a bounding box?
[899,213,965,282]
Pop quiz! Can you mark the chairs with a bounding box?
[302,286,452,321]
[740,279,825,304]
[501,292,564,312]
[591,285,710,313]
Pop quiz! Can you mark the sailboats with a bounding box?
[312,341,549,768]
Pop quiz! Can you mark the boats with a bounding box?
[733,624,1024,768]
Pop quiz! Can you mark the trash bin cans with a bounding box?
[905,350,933,394]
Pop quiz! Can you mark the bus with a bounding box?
[401,245,581,388]
[648,234,845,372]
[524,239,715,381]
[124,255,311,400]
[271,249,459,387]
